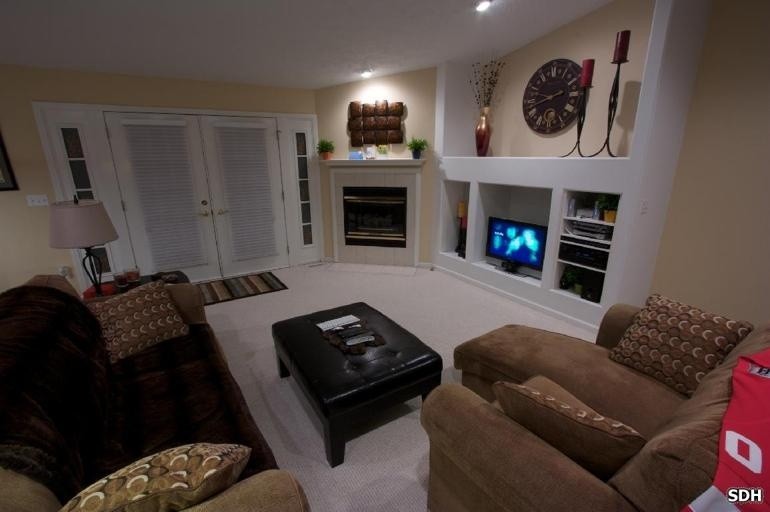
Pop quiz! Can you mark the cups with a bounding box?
[113,272,129,287]
[124,266,141,282]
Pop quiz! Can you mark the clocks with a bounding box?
[521,58,589,137]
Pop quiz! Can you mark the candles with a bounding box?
[612,29,632,62]
[580,61,596,86]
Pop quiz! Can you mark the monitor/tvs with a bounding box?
[486,216,548,280]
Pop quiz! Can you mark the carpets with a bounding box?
[195,270,287,307]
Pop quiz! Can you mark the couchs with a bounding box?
[0,270,312,512]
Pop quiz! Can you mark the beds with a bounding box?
[271,300,446,469]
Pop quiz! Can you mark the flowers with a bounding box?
[466,56,508,107]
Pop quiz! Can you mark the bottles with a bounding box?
[458,190,465,218]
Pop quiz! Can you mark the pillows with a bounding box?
[610,291,756,401]
[55,438,253,511]
[84,276,195,363]
[489,374,651,482]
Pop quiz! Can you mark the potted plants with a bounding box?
[316,137,334,160]
[406,137,429,159]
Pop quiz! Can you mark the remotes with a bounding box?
[338,326,376,347]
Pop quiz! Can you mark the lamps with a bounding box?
[46,192,120,297]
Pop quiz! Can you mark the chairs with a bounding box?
[419,301,770,510]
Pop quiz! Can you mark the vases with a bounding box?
[473,103,492,155]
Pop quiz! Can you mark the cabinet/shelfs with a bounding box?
[557,214,613,274]
